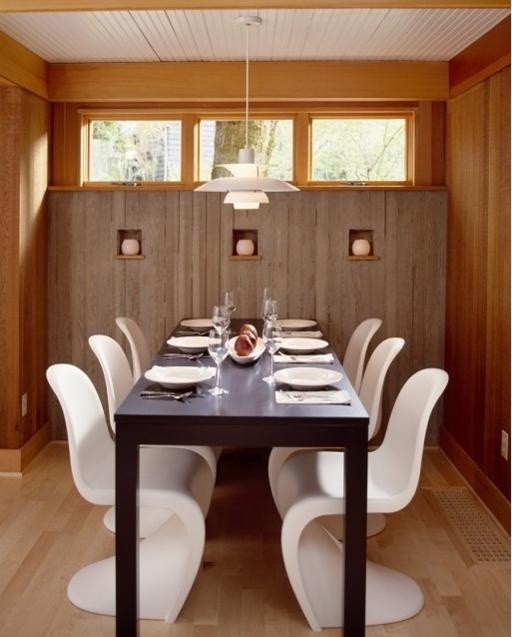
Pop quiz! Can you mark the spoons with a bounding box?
[141,391,193,400]
[162,353,204,360]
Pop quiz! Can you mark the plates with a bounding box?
[167,333,221,354]
[274,366,343,391]
[142,363,217,390]
[269,317,316,331]
[274,337,329,353]
[178,316,221,332]
[226,336,265,364]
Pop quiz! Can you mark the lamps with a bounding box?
[193,14,301,210]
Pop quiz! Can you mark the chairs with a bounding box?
[45,362,214,624]
[273,367,450,633]
[268,336,405,540]
[342,318,383,394]
[115,316,152,384]
[88,335,217,520]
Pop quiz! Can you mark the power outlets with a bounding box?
[501,430,510,459]
[21,393,29,417]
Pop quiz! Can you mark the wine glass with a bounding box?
[263,329,280,383]
[208,330,229,396]
[221,289,237,315]
[213,305,230,343]
[262,300,278,323]
[263,288,277,308]
